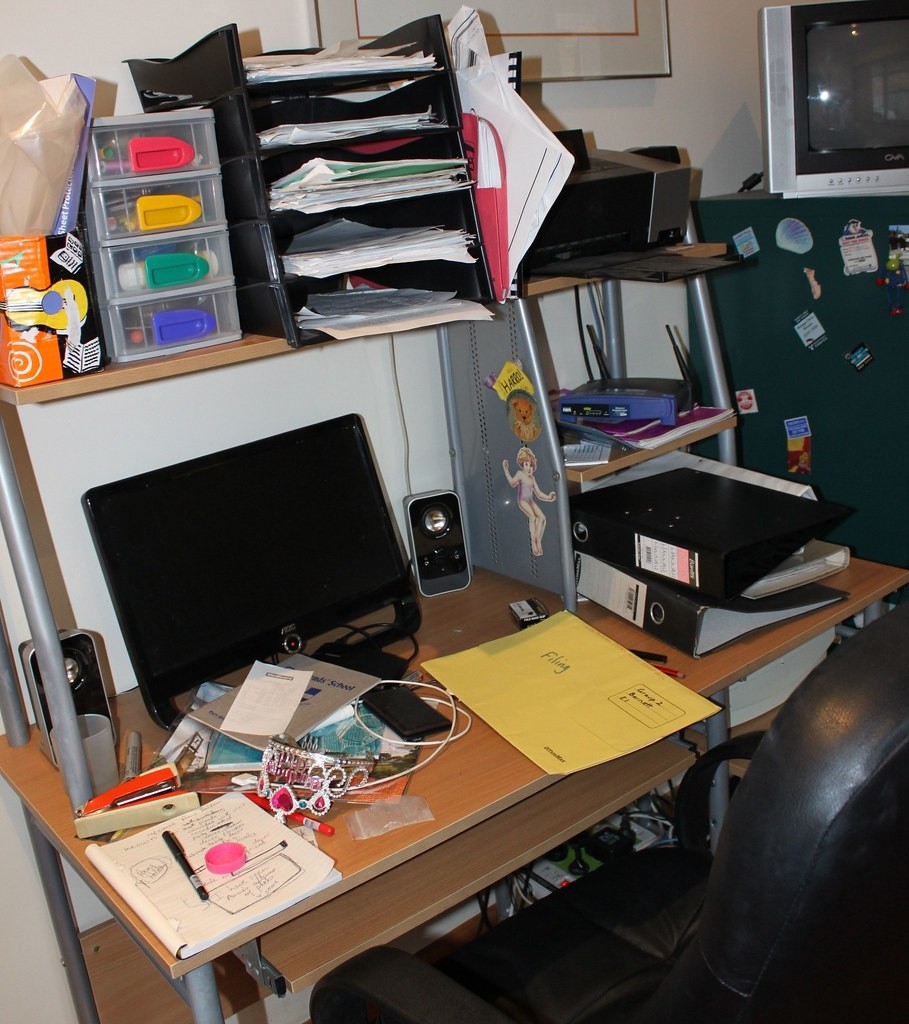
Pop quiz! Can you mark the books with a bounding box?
[187,654,385,767]
[83,789,345,962]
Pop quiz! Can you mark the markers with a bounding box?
[122,731,142,781]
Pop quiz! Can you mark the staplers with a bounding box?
[71,761,201,841]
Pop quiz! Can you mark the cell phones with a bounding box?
[362,686,452,743]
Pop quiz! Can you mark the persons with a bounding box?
[888,230,906,256]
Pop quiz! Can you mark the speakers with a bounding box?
[403,490,470,597]
[19,628,118,766]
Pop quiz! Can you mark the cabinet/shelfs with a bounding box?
[0,147,738,813]
[122,14,496,349]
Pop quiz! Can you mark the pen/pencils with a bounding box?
[651,664,686,678]
[629,650,669,663]
[160,830,209,901]
[240,792,335,837]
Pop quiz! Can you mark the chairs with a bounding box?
[310,601,909,1024]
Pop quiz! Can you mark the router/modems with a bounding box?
[558,324,694,427]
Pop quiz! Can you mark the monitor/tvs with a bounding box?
[758,0,909,198]
[80,413,421,729]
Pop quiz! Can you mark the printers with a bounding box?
[519,139,693,271]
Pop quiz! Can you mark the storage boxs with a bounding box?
[80,109,243,364]
[0,229,106,388]
[38,73,96,235]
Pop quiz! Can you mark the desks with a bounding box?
[690,188,909,570]
[0,560,909,1024]
